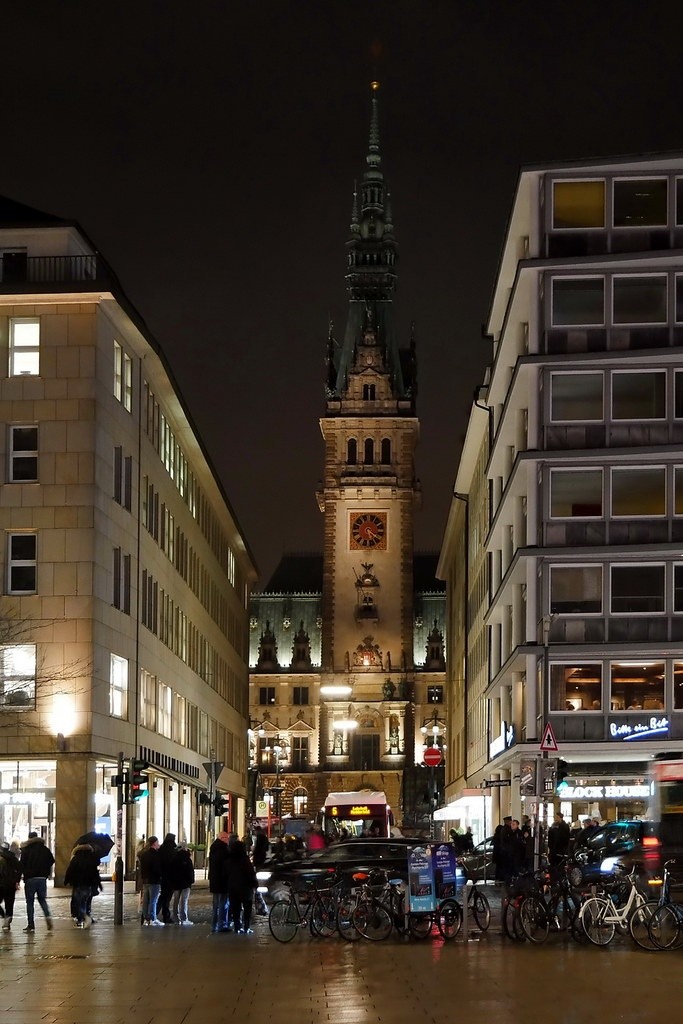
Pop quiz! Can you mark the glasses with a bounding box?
[221,836,230,839]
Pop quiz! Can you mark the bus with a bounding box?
[259,820,314,845]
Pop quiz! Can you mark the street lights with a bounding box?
[265,747,292,817]
[270,786,285,818]
[422,726,447,809]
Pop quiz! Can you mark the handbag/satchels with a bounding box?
[91,869,102,897]
[255,890,268,916]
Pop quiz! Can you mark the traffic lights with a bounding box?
[198,790,209,805]
[216,789,230,816]
[129,758,150,802]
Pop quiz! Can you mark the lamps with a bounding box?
[421,710,447,749]
[244,718,291,753]
[316,617,322,628]
[57,732,65,752]
[169,785,172,791]
[249,616,257,629]
[414,615,424,627]
[283,617,290,630]
[183,789,186,794]
[153,782,157,787]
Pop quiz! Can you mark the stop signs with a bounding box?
[423,748,441,766]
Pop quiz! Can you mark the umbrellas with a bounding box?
[71,831,114,859]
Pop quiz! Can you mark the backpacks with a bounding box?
[2,852,22,886]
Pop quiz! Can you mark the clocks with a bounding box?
[348,510,389,552]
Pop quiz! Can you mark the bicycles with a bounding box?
[268,868,683,953]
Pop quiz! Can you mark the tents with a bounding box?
[324,791,387,838]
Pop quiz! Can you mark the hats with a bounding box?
[1,842,10,849]
[591,817,602,825]
[503,816,512,820]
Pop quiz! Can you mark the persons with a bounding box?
[14,832,55,931]
[572,816,601,852]
[241,825,270,873]
[566,697,641,710]
[0,840,22,932]
[63,843,103,930]
[272,821,381,856]
[449,826,474,856]
[208,831,259,935]
[545,811,571,886]
[490,812,544,886]
[136,832,196,926]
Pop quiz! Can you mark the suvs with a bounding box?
[567,815,683,899]
[256,837,442,905]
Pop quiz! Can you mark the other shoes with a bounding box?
[182,920,194,925]
[2,924,11,930]
[244,928,253,933]
[83,916,92,929]
[175,921,182,925]
[163,918,174,923]
[150,919,164,926]
[236,927,245,934]
[212,930,219,934]
[73,921,84,928]
[4,917,12,925]
[142,918,150,926]
[218,927,233,933]
[46,919,53,931]
[23,924,35,931]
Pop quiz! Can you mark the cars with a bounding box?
[455,834,497,882]
